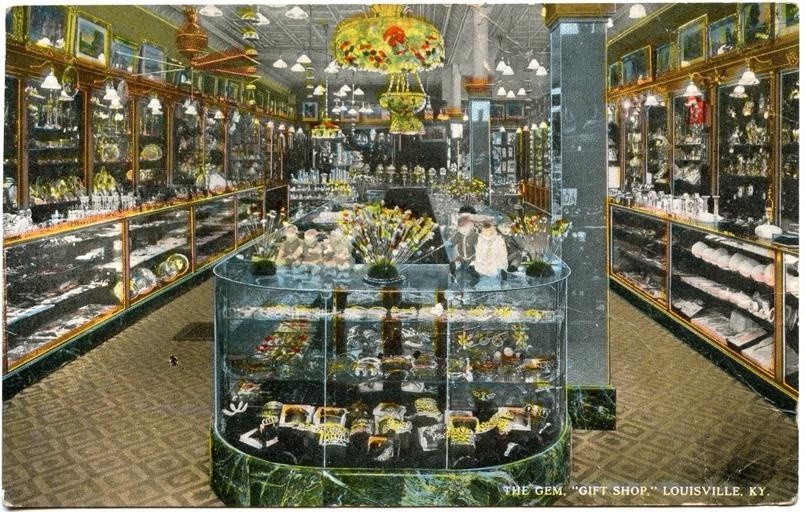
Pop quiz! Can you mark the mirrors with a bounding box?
[549,18,610,387]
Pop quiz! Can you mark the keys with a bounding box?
[444,213,525,292]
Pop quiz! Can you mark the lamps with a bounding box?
[644,89,658,106]
[516,121,548,134]
[285,7,365,98]
[267,119,304,134]
[331,4,445,136]
[683,75,704,97]
[496,44,551,98]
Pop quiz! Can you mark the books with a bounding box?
[720,182,768,236]
[275,222,356,280]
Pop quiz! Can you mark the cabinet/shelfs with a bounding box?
[2,179,266,374]
[607,190,799,404]
[213,185,570,470]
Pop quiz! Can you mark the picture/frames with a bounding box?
[340,105,383,124]
[5,5,296,121]
[609,1,800,92]
[418,126,447,143]
[302,101,319,121]
[504,102,526,120]
[490,105,505,121]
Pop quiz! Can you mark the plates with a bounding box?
[754,225,783,238]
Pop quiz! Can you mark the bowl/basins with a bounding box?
[114,253,192,301]
